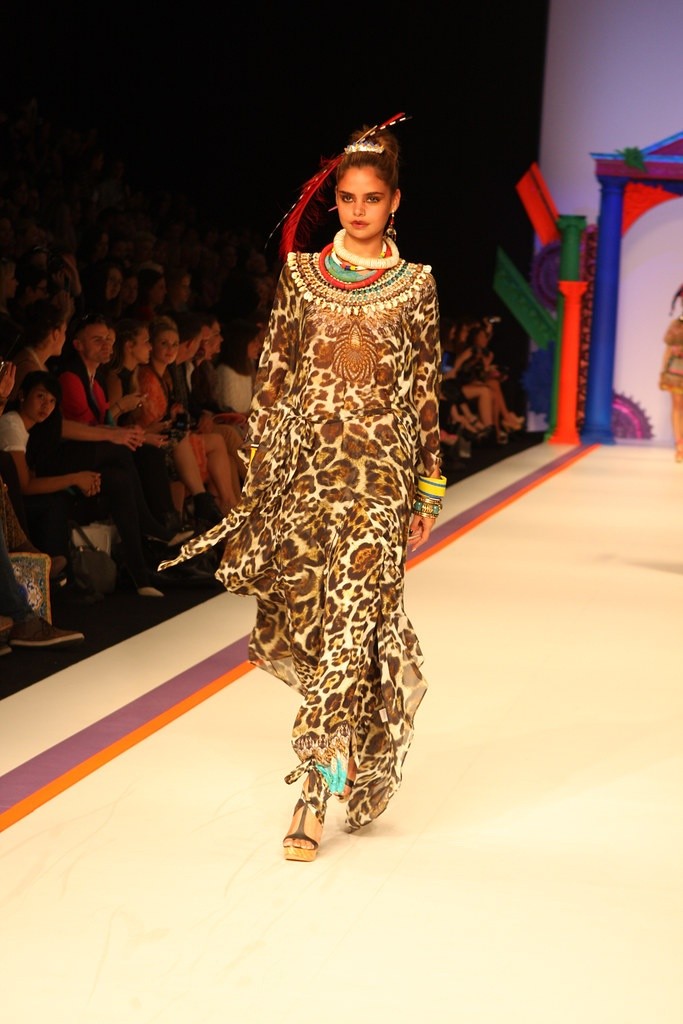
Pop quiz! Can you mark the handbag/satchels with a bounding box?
[63,521,117,596]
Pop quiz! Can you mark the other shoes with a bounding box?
[495,432,509,446]
[79,593,104,605]
[49,556,66,577]
[440,429,458,445]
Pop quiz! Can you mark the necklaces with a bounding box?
[318,228,401,289]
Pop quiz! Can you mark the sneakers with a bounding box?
[0,643,12,656]
[9,617,85,648]
[193,492,222,523]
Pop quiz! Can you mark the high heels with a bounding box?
[333,754,354,802]
[121,560,165,599]
[501,413,525,433]
[145,530,195,547]
[282,796,323,863]
[462,420,490,439]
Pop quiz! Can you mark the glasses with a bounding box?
[81,312,103,322]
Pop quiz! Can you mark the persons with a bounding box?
[1,191,528,659]
[658,284,683,461]
[212,125,451,865]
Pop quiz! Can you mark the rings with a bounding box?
[137,401,144,408]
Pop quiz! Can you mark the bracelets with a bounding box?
[115,402,125,413]
[410,473,447,520]
[0,397,8,406]
